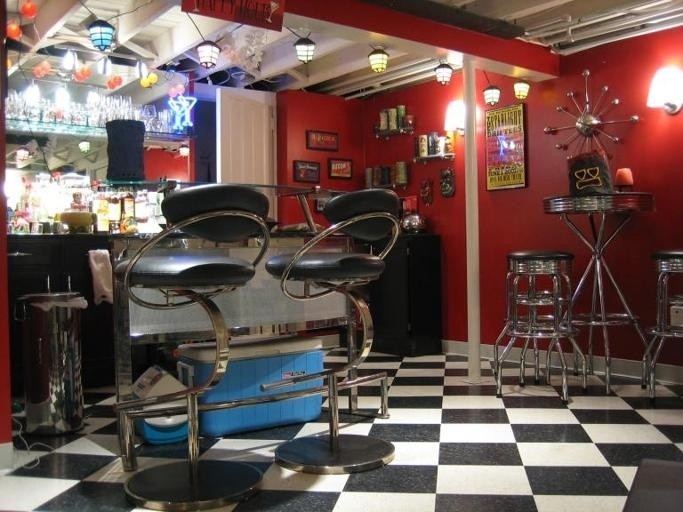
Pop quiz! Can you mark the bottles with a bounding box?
[2,170,164,233]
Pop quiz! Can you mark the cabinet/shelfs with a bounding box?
[56,236,115,392]
[6,233,59,401]
[351,232,447,359]
[360,128,456,194]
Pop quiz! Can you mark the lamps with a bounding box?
[17,145,30,162]
[179,143,190,157]
[79,136,91,154]
[645,63,683,119]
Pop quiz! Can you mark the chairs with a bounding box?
[108,182,272,512]
[266,187,405,478]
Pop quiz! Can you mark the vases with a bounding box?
[306,128,340,152]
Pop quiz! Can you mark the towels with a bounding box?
[89,249,115,307]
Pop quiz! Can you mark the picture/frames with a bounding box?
[293,159,321,184]
[327,158,354,181]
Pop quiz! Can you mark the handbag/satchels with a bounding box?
[568,149,612,196]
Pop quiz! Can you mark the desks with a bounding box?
[542,191,660,394]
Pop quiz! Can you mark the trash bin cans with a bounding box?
[12,292,86,438]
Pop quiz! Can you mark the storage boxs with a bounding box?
[176,338,329,439]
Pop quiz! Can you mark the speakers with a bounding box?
[107,118,146,181]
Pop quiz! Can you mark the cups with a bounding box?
[3,80,174,134]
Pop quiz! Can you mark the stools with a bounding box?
[493,247,590,402]
[640,248,683,398]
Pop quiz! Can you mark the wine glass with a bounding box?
[168,94,198,131]
